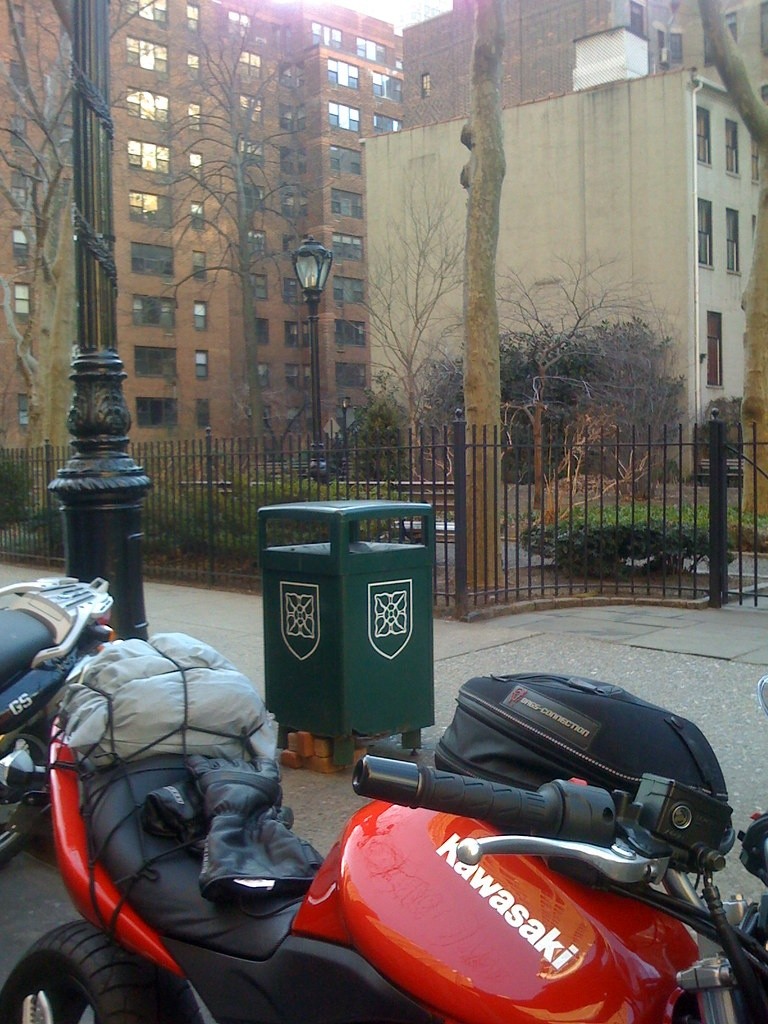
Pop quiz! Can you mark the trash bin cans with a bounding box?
[254,501,437,767]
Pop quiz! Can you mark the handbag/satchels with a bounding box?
[435,672,732,828]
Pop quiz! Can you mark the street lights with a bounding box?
[341,396,349,451]
[292,238,333,476]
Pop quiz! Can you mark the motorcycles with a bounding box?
[0,575,116,866]
[0,630,765,1024]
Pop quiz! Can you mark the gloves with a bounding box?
[141,776,325,869]
[184,754,315,903]
